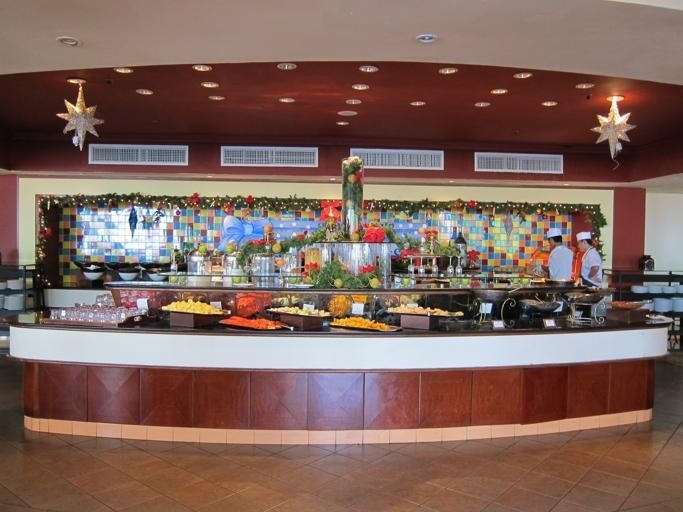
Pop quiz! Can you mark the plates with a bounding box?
[233,282,253,287]
[630,284,683,312]
[294,283,314,288]
[607,300,644,309]
[0,279,25,310]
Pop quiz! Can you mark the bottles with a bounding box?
[449,225,467,255]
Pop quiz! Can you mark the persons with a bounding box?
[576,231,604,288]
[539,226,574,280]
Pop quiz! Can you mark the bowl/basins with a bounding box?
[118,272,138,281]
[148,271,168,281]
[81,270,105,280]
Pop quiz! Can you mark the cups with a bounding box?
[47,292,149,323]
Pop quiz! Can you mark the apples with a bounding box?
[233,277,241,284]
[199,246,207,253]
[288,278,297,284]
[403,278,410,286]
[264,225,272,233]
[170,277,177,282]
[346,201,354,207]
[371,278,380,288]
[225,246,234,254]
[462,279,469,285]
[277,259,283,264]
[272,244,281,253]
[348,175,356,182]
[335,279,342,288]
[452,278,458,283]
[513,272,530,285]
[351,233,359,241]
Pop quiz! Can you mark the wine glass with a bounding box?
[444,255,464,277]
[416,254,427,276]
[430,255,438,274]
[406,254,416,277]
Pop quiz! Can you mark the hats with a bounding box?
[576,231,591,241]
[546,228,562,239]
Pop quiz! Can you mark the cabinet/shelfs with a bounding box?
[602,271,683,351]
